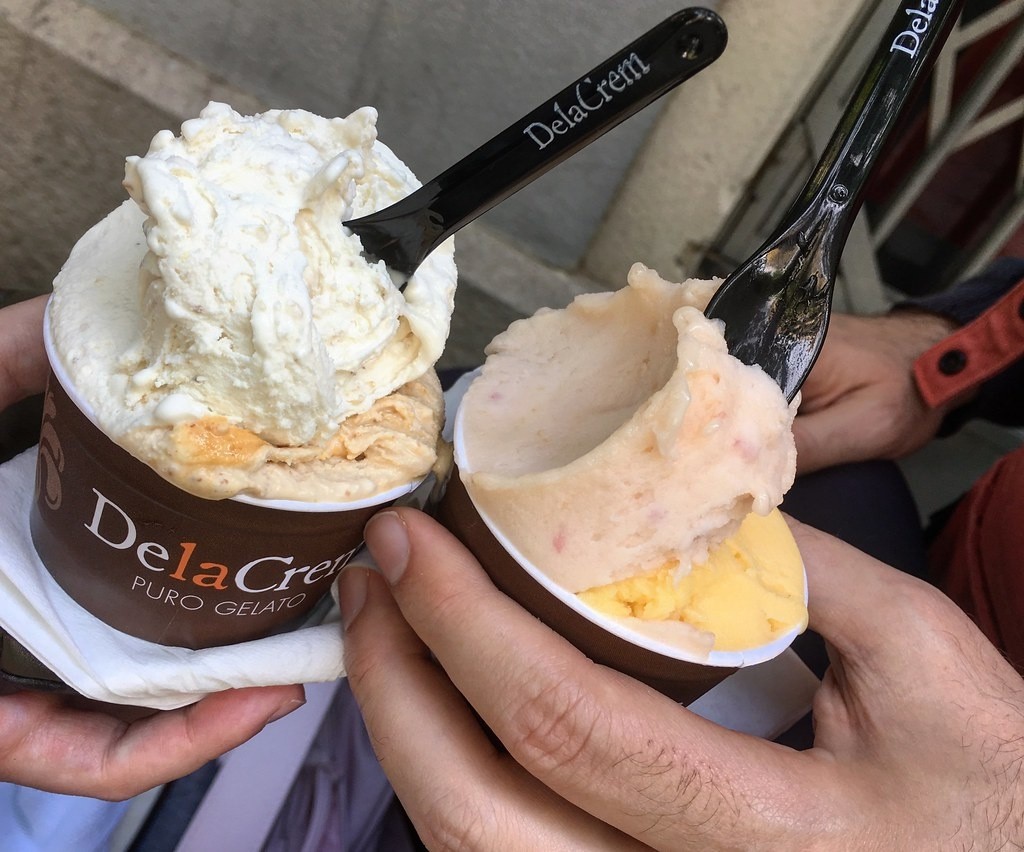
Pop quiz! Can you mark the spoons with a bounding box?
[701,0,966,403]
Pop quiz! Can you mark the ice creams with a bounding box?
[454,263,810,657]
[46,101,460,503]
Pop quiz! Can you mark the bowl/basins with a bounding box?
[422,456,808,709]
[28,292,428,651]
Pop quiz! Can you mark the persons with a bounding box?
[338,255,1024,852]
[0,291,307,852]
